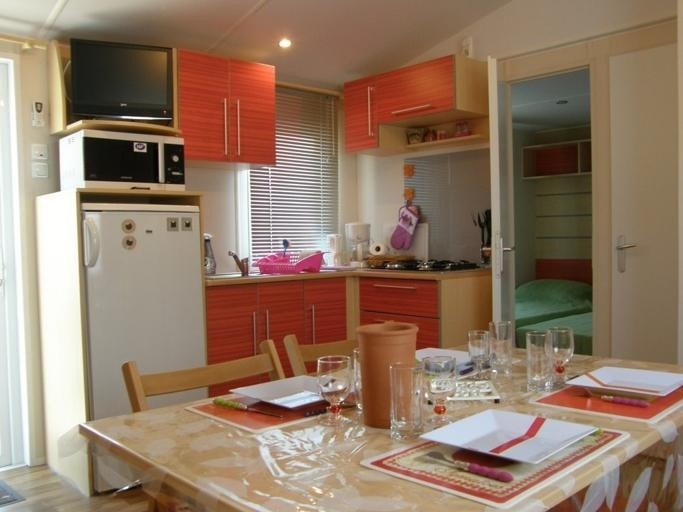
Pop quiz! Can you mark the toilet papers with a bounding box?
[371,243,388,256]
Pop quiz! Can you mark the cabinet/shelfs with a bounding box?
[178,48,276,172]
[206,279,304,398]
[358,276,491,351]
[378,54,489,159]
[522,140,591,179]
[342,75,407,155]
[45,37,178,134]
[304,275,347,373]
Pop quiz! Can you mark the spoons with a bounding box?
[415,451,512,482]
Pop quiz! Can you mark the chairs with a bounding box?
[284,335,360,376]
[122,340,285,411]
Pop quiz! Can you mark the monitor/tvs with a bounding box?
[70,38,172,121]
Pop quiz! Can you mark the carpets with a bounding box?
[0,476,26,509]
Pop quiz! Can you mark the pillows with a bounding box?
[517,279,591,303]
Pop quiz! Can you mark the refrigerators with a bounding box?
[81,203,208,494]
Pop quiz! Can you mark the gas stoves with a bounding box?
[385,258,480,271]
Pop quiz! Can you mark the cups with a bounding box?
[353,321,553,440]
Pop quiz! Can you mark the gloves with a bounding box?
[390,205,419,251]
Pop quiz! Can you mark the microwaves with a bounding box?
[60,129,185,191]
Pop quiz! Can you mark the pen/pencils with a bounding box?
[460,367,473,375]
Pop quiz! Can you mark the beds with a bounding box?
[515,258,593,327]
[517,313,593,353]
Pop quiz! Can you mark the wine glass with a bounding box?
[316,328,575,427]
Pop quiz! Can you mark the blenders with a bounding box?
[327,234,346,266]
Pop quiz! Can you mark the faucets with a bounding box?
[227,250,250,277]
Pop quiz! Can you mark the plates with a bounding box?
[566,362,683,398]
[228,375,352,408]
[412,345,502,380]
[415,408,600,468]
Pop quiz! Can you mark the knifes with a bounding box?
[213,398,283,417]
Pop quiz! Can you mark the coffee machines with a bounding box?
[345,223,371,268]
